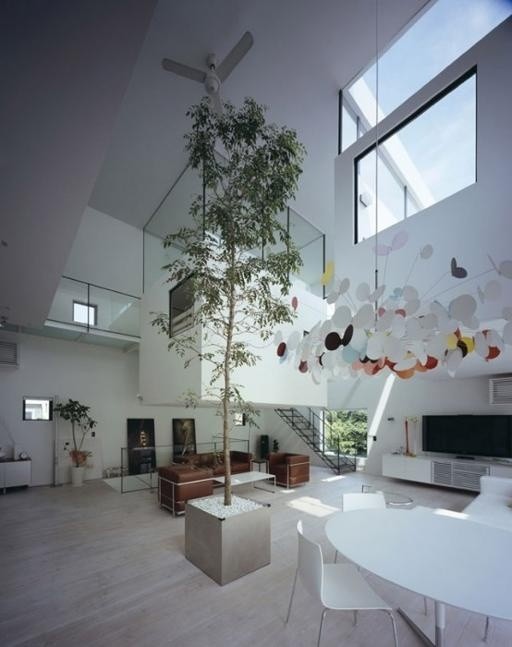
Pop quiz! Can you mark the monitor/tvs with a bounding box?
[422,414,512,459]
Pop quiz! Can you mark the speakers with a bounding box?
[260,435,269,460]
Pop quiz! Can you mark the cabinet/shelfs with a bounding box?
[0,460,32,494]
[381,453,512,492]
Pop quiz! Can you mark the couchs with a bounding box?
[173,450,252,487]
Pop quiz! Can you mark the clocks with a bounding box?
[19,452,28,460]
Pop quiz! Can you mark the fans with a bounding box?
[162,31,254,117]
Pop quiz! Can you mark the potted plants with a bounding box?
[53,398,97,486]
[150,95,309,584]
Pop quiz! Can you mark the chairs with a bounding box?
[334,493,427,627]
[284,520,398,647]
[159,465,214,516]
[458,476,512,641]
[268,452,310,489]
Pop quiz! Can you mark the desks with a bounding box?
[324,507,512,647]
